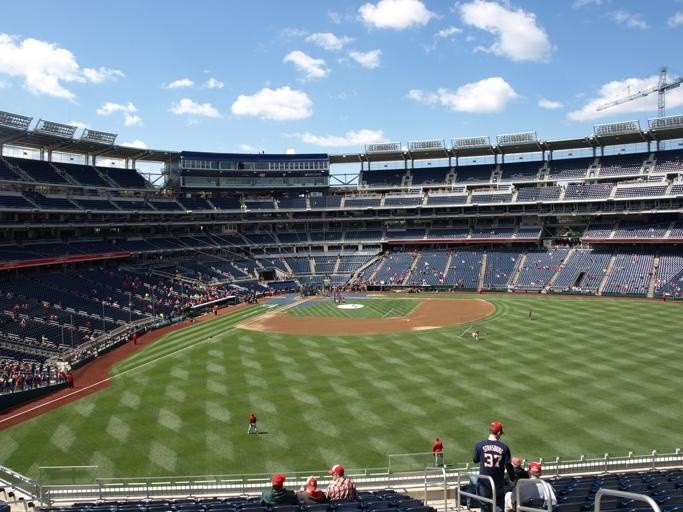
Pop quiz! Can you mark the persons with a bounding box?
[297,475,327,504]
[473,421,516,512]
[247,412,259,434]
[504,460,555,511]
[260,473,297,505]
[504,457,530,485]
[326,464,357,502]
[432,437,444,467]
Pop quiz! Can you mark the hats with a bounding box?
[490,421,506,435]
[528,462,542,475]
[305,476,318,486]
[512,456,522,466]
[327,464,345,476]
[272,474,286,485]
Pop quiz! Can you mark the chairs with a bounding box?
[35,488,438,512]
[194,147,683,298]
[0,152,195,392]
[456,467,682,512]
